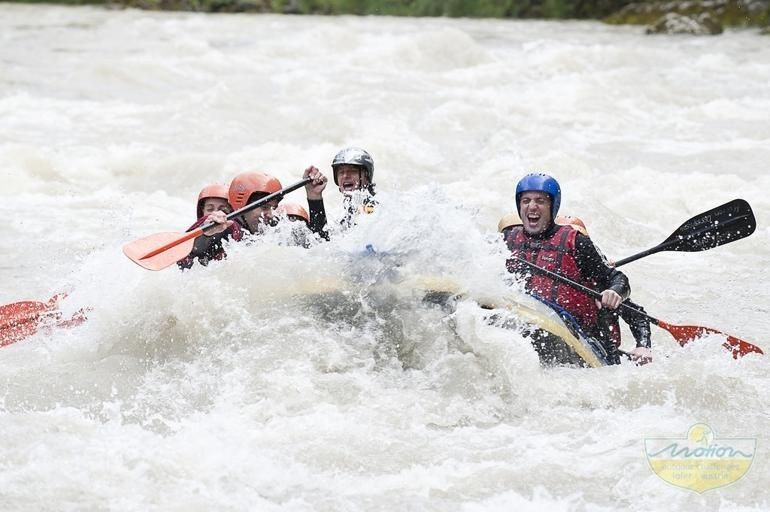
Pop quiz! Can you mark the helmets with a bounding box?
[553,215,590,239]
[497,213,523,233]
[275,201,310,225]
[331,147,375,189]
[196,183,235,224]
[228,171,284,214]
[515,173,561,224]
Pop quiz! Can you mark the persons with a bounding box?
[500,172,652,366]
[176,146,379,271]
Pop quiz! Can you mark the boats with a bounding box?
[52,244,613,371]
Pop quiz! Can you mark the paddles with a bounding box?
[607,199,755,269]
[123,178,324,270]
[512,257,765,362]
[0,302,57,346]
